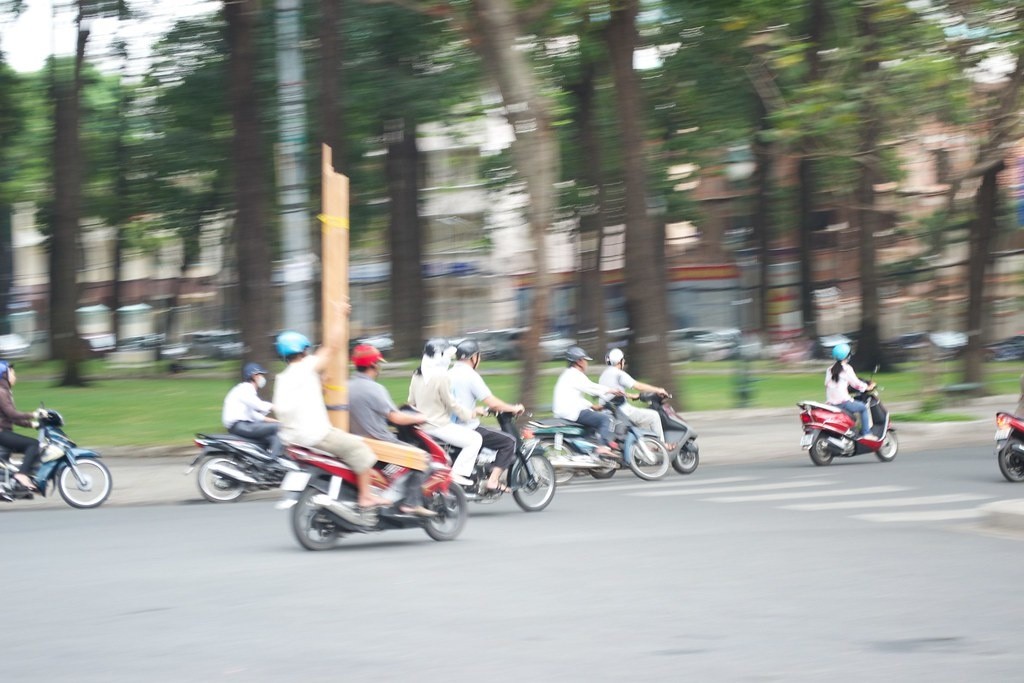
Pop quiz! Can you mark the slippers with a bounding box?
[361,497,393,511]
[489,483,512,493]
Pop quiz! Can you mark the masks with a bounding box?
[255,376,266,389]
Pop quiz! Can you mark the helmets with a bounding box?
[566,346,593,362]
[605,348,623,365]
[455,340,481,359]
[424,337,451,357]
[276,331,311,356]
[0,360,14,380]
[353,346,387,366]
[832,344,850,361]
[243,363,269,379]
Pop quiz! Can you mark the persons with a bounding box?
[273,296,395,511]
[1015,373,1024,417]
[553,347,625,458]
[407,338,483,484]
[0,362,48,489]
[349,345,439,515]
[825,344,878,440]
[449,340,525,494]
[222,364,287,470]
[598,349,678,450]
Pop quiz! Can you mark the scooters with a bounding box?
[796,364,899,466]
[590,391,700,479]
[993,411,1024,483]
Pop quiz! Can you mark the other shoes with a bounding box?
[12,474,37,489]
[863,434,879,441]
[400,504,435,516]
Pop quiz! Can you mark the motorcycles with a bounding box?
[525,388,671,487]
[185,432,306,504]
[276,404,467,553]
[0,402,113,508]
[420,404,557,513]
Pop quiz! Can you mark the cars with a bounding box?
[0,325,249,366]
[348,314,1024,370]
